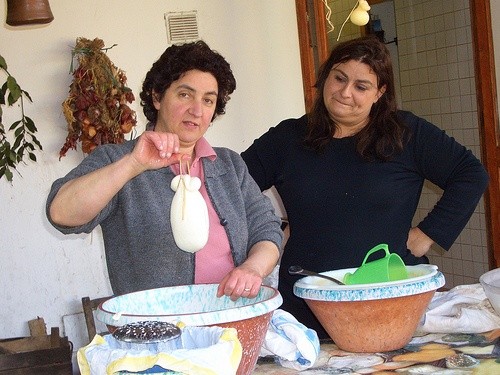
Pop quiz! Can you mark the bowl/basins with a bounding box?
[96,283,283,375]
[293,264,446,352]
[478,267,500,316]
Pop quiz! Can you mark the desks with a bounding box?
[254,333,500,375]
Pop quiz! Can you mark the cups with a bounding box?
[343,244,409,284]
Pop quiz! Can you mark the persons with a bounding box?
[45,40,284,301]
[239,37,488,340]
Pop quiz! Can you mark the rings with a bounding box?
[245,288,250,291]
